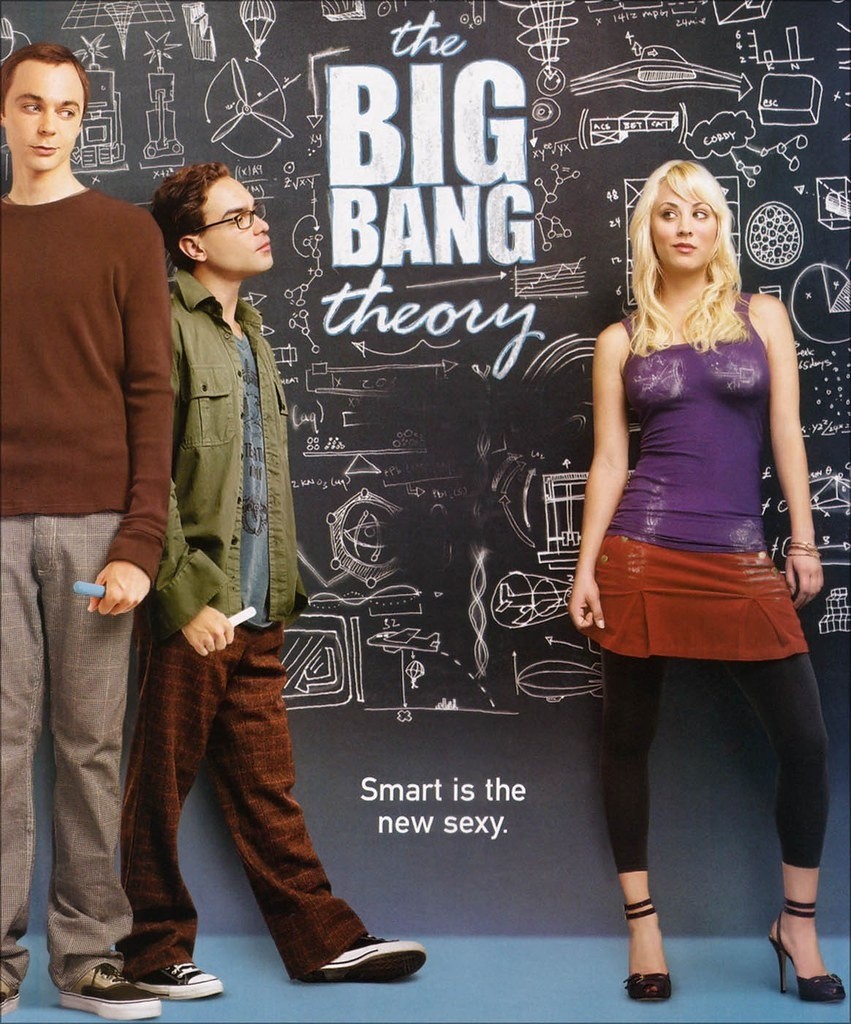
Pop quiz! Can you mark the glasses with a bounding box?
[191,197,266,235]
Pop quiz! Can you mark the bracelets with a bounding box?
[786,541,822,560]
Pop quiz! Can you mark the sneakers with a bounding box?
[297,931,425,984]
[0,979,20,1017]
[60,963,162,1020]
[131,962,224,1001]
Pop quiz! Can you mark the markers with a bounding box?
[72,579,105,598]
[227,607,256,626]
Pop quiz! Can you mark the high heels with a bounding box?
[623,896,674,1001]
[766,897,844,1005]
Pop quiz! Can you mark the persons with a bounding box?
[569,160,847,1003]
[0,42,176,1020]
[115,163,428,1000]
